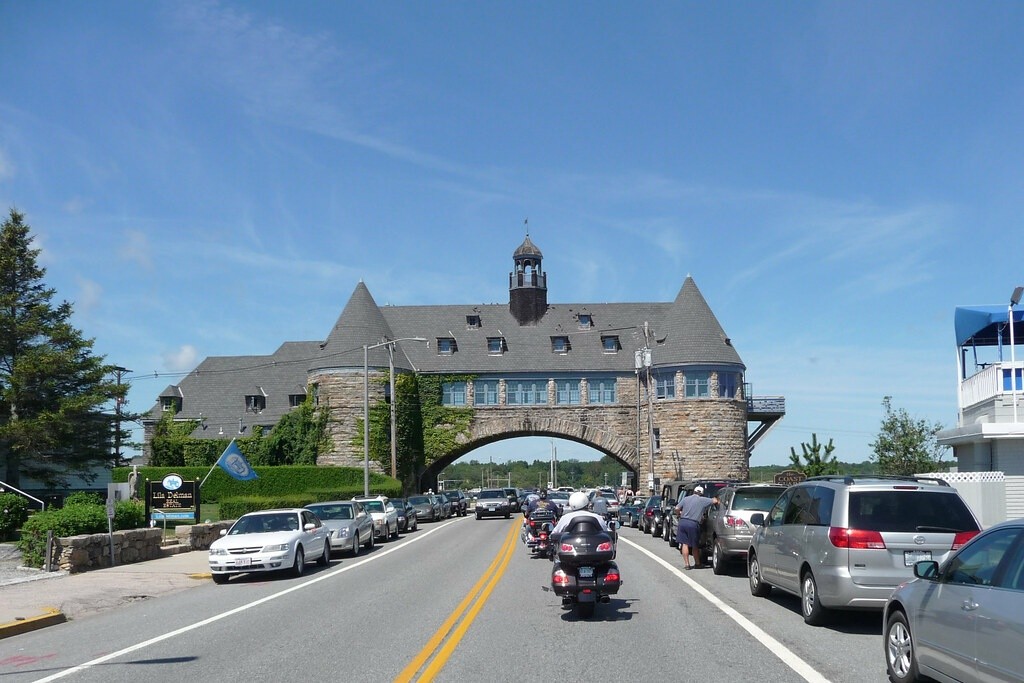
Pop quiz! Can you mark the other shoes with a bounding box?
[694,564,705,569]
[685,565,690,570]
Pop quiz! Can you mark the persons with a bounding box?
[613,485,658,504]
[549,491,608,535]
[523,487,611,517]
[675,485,719,570]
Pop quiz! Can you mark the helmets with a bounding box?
[568,492,588,511]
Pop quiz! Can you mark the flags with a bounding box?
[217,441,257,481]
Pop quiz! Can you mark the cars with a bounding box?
[390,498,418,533]
[304,501,375,557]
[882,518,1024,683]
[503,488,525,512]
[435,494,453,520]
[208,508,333,584]
[408,495,441,523]
[521,486,621,516]
[349,495,400,543]
[618,496,662,534]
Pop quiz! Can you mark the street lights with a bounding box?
[364,338,429,497]
[437,472,445,493]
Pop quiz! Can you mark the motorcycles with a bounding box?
[541,516,622,607]
[521,503,564,559]
[589,505,612,526]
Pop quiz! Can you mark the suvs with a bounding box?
[650,478,749,555]
[699,483,790,576]
[438,489,467,517]
[473,488,512,521]
[747,474,988,625]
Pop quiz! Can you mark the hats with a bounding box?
[694,486,704,494]
